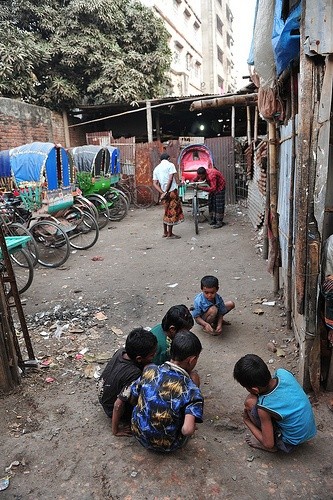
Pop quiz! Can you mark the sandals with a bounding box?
[163,233,178,239]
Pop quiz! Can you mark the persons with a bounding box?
[191,167,225,229]
[322,234,333,412]
[233,354,317,453]
[96,303,203,454]
[153,152,185,240]
[192,275,235,335]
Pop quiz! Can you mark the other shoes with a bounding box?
[210,221,223,229]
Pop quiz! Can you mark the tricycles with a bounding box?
[0,141,131,299]
[177,143,215,235]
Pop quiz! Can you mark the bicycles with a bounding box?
[121,173,154,209]
